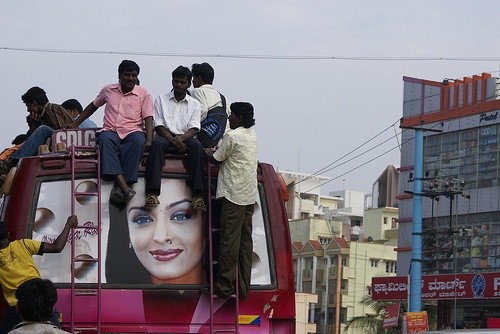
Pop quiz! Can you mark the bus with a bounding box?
[1,126,298,334]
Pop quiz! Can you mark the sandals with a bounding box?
[110,181,136,203]
[192,63,214,85]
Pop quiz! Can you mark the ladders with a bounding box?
[206,162,238,334]
[68,144,101,334]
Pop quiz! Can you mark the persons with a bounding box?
[201,102,258,300]
[145,65,208,212]
[9,278,73,334]
[106,177,222,285]
[65,59,153,203]
[0,215,78,329]
[0,86,79,173]
[61,99,97,129]
[190,62,231,148]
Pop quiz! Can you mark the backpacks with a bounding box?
[196,91,228,148]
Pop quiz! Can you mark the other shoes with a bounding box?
[191,195,207,212]
[145,194,160,207]
